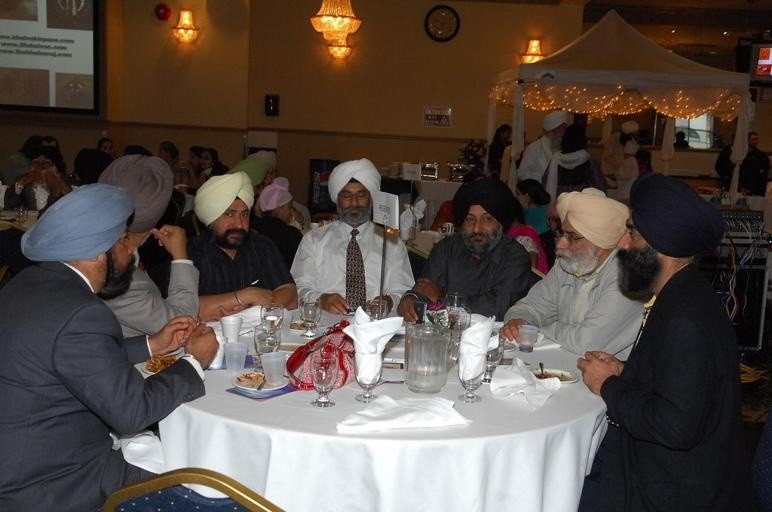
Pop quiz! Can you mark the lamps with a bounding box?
[310,0,362,59]
[520,39,546,64]
[172,8,199,44]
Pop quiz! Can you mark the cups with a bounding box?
[253,325,281,355]
[204,335,224,369]
[432,221,456,247]
[259,302,284,329]
[221,342,248,375]
[366,299,389,320]
[309,222,319,231]
[13,206,29,225]
[258,352,286,387]
[220,316,242,343]
[518,323,538,353]
[403,322,458,394]
[482,337,505,385]
[447,291,472,329]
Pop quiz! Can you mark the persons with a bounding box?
[715,130,770,197]
[2,135,307,511]
[673,131,689,150]
[500,187,645,379]
[396,177,530,325]
[290,158,416,320]
[577,169,758,510]
[488,111,654,274]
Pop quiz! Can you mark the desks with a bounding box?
[380,178,466,231]
[741,194,772,234]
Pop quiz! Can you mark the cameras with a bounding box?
[345,306,368,316]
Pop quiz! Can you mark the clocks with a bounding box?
[424,4,460,43]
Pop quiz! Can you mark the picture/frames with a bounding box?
[0,0,101,114]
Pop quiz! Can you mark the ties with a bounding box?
[344,228,367,309]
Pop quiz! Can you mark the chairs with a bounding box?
[523,265,546,297]
[313,212,339,222]
[92,468,283,511]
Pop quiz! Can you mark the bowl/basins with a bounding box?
[381,162,401,179]
[698,194,713,202]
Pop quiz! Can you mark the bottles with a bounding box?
[713,188,721,202]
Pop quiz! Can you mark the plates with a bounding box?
[276,343,300,354]
[502,342,516,352]
[0,220,13,232]
[534,369,582,384]
[287,320,319,335]
[228,370,289,394]
[140,361,159,376]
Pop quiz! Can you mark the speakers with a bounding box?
[265,94,280,116]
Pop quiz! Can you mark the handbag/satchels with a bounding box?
[288,319,364,391]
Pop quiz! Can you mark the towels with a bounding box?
[196,303,266,369]
[489,356,562,412]
[399,198,427,241]
[342,305,404,384]
[458,314,497,382]
[335,393,471,432]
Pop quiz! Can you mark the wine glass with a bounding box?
[308,350,339,408]
[459,362,485,404]
[352,350,384,405]
[299,296,322,339]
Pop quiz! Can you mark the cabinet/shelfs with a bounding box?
[675,175,720,194]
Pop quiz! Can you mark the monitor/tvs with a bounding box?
[749,43,772,83]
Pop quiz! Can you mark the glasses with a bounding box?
[553,228,585,241]
